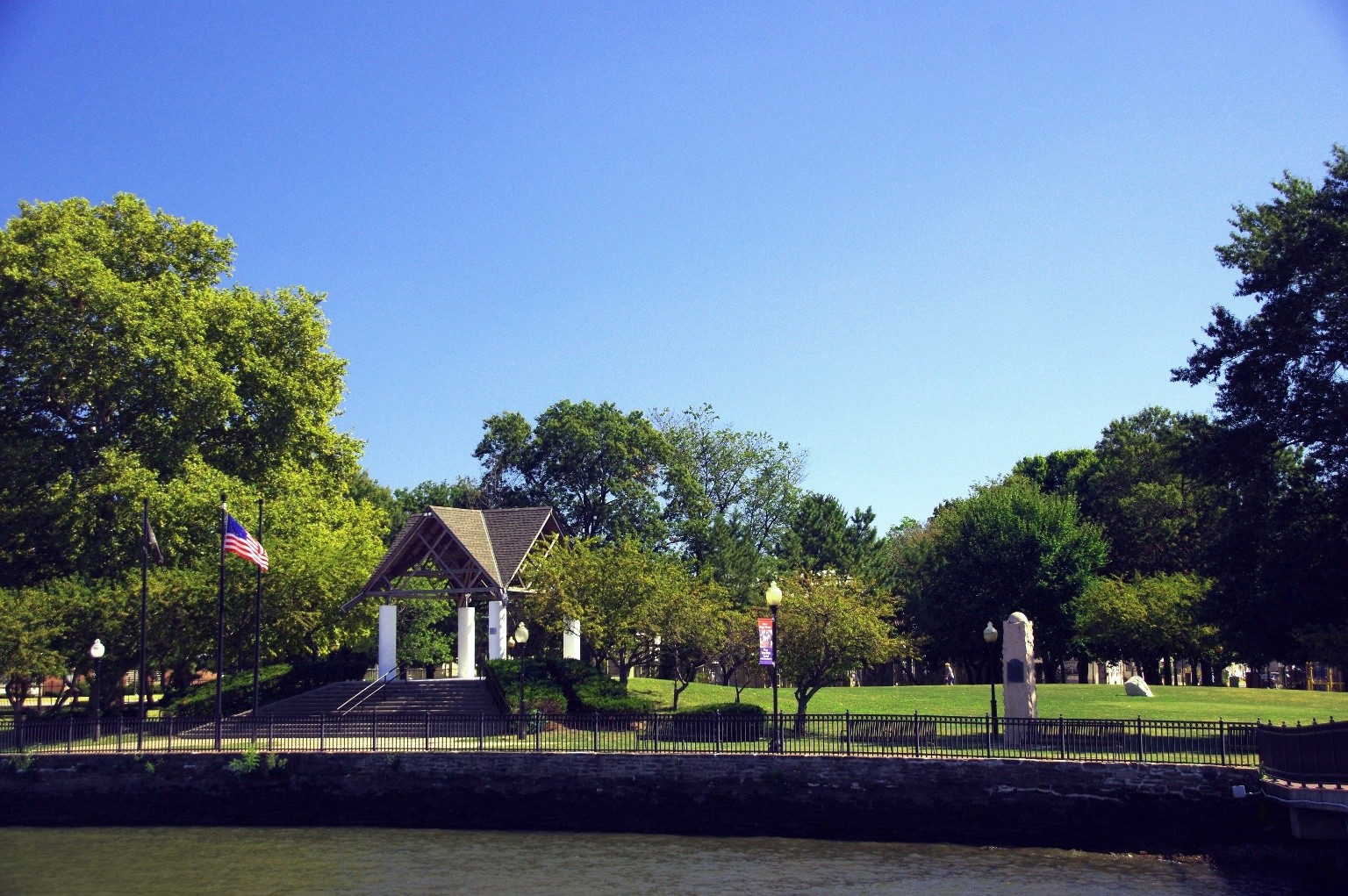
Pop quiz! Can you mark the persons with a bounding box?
[853,676,859,686]
[945,662,954,685]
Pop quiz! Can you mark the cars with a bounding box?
[1260,674,1278,688]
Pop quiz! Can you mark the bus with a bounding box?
[694,661,723,684]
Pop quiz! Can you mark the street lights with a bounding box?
[508,636,515,659]
[983,621,1000,744]
[765,581,784,750]
[514,622,530,740]
[89,638,106,743]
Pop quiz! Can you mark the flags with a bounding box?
[141,511,163,566]
[221,503,269,573]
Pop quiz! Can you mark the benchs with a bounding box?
[841,720,936,755]
[1019,720,1125,760]
[635,718,727,754]
[1210,727,1258,753]
